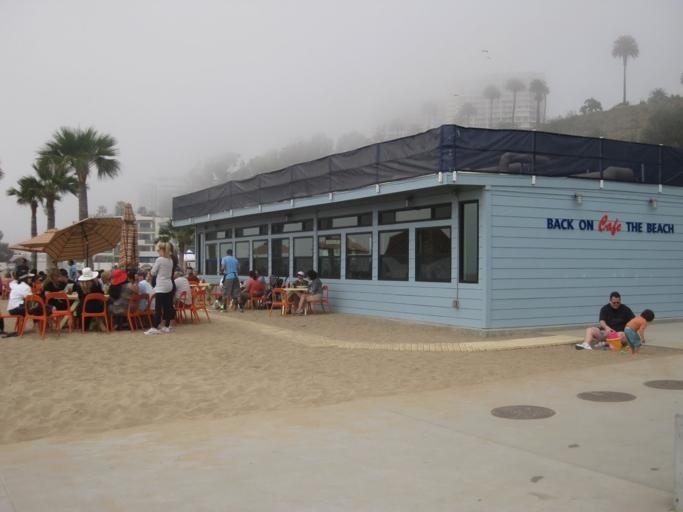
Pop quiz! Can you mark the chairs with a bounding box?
[205,281,332,316]
[0,277,210,340]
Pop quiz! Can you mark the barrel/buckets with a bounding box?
[606,335,623,352]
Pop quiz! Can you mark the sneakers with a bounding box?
[576,342,592,350]
[144,328,159,335]
[159,327,171,332]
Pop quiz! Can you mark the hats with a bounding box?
[297,271,304,276]
[77,267,99,281]
[111,269,128,285]
[18,271,35,280]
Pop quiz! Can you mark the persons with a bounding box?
[220,249,243,312]
[623,309,655,357]
[574,291,636,350]
[3,239,199,337]
[220,270,327,318]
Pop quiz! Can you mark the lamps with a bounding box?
[570,190,583,206]
[645,196,659,210]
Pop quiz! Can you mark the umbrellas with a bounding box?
[43,215,124,268]
[119,201,140,277]
[18,228,59,246]
